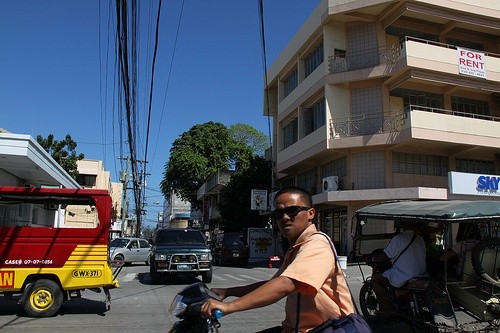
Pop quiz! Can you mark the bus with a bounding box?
[0,186,121,318]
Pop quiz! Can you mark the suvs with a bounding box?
[148,227,212,283]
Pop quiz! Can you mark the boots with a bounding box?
[375,287,395,317]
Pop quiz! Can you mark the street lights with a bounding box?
[141,200,160,235]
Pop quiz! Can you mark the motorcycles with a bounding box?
[347,199,499,333]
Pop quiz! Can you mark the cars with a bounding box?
[107,237,153,267]
[208,231,250,268]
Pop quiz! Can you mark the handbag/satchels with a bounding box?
[306,313,381,333]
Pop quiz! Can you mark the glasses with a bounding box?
[271,206,311,220]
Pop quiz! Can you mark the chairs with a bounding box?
[159,231,173,242]
[456,249,500,281]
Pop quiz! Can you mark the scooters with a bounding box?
[168,282,284,333]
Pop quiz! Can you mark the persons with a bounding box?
[373,221,427,316]
[201,186,356,332]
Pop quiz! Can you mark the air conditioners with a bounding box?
[321,175,339,193]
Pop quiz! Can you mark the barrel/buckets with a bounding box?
[338,256,347,270]
